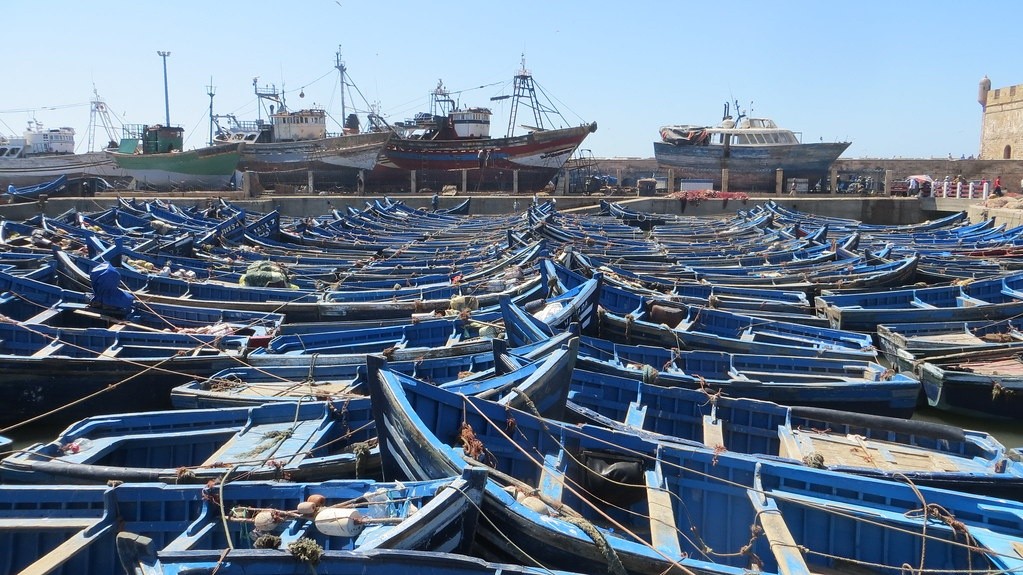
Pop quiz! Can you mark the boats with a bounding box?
[0,81,147,199]
[653,98,853,193]
[489,337,1023,504]
[101,49,247,192]
[0,170,1023,575]
[211,44,416,195]
[378,50,598,195]
[364,351,1023,575]
[1,173,70,203]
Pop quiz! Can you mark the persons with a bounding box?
[7,183,16,204]
[944,175,1002,197]
[432,192,439,213]
[207,204,224,218]
[478,148,487,169]
[788,174,883,197]
